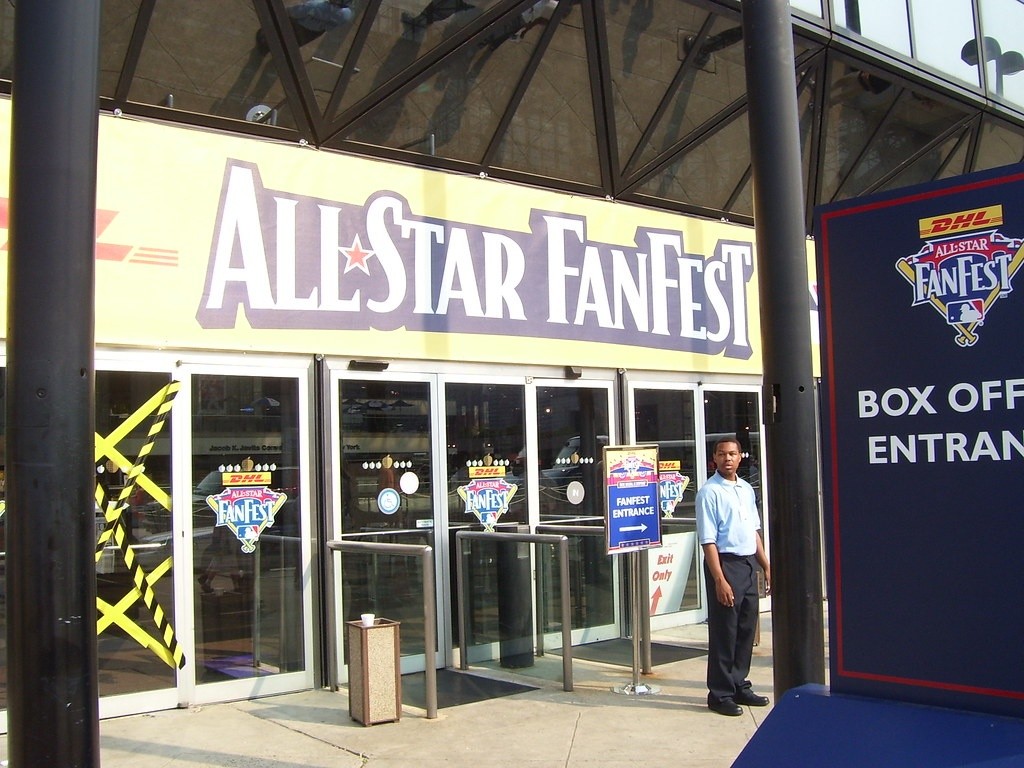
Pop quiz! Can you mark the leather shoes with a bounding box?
[707,695,743,716]
[734,690,770,706]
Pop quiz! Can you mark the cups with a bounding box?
[361,614,375,626]
[216,588,224,596]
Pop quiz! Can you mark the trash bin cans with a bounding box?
[344,616,402,727]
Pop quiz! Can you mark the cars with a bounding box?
[103,497,360,582]
[357,465,527,524]
[140,462,358,532]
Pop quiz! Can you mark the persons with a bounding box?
[694,436,770,717]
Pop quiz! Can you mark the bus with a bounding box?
[553,434,761,501]
